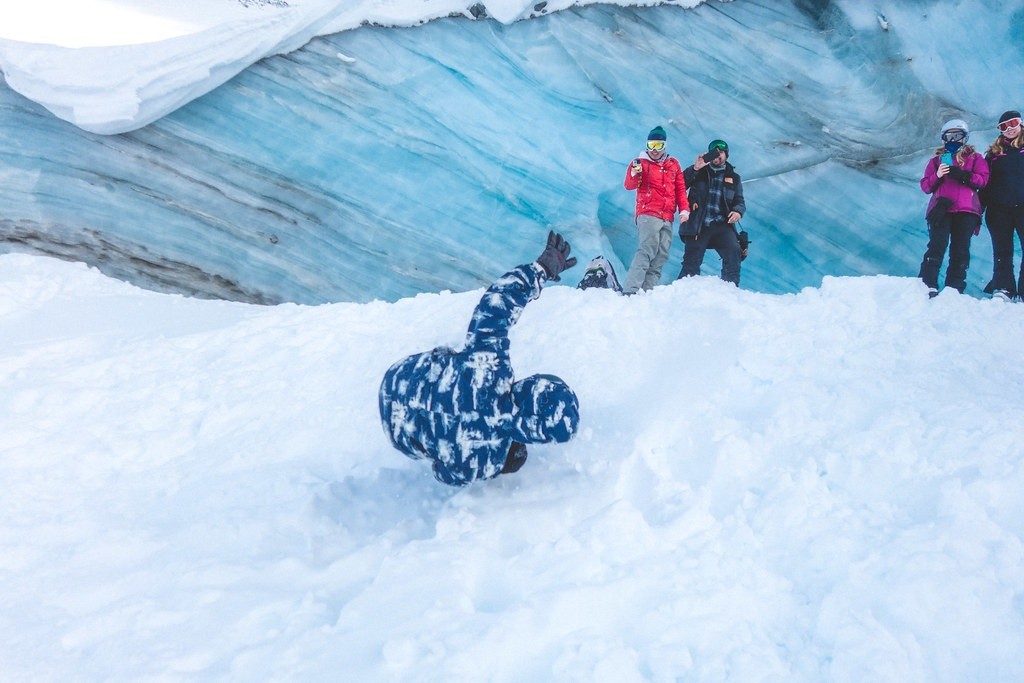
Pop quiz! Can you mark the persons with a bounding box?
[677,140,746,290]
[376,229,582,489]
[970,110,1024,305]
[920,119,990,298]
[620,126,690,297]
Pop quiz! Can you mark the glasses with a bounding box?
[647,141,666,150]
[996,117,1021,132]
[943,131,966,143]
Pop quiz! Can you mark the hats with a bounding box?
[648,125,667,141]
[998,111,1022,123]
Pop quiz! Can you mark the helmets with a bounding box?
[708,139,729,158]
[939,118,970,144]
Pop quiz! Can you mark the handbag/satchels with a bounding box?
[738,231,751,262]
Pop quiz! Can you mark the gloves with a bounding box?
[535,232,577,282]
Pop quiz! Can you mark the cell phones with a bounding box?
[940,153,953,167]
[702,147,720,164]
[632,159,640,167]
